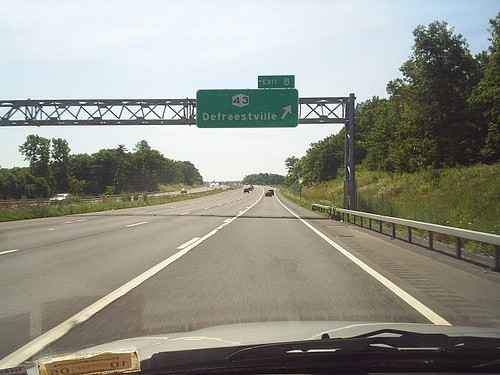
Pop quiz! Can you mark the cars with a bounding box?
[181,187,187,193]
[49,192,73,205]
[244,185,275,197]
